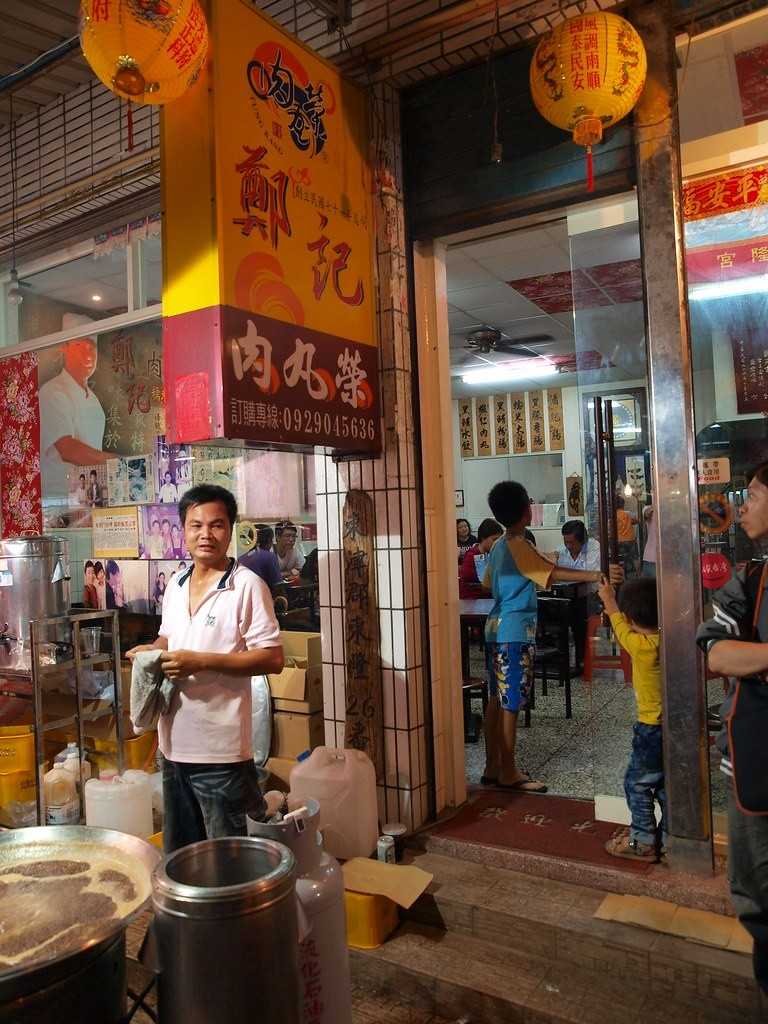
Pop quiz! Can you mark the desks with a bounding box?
[289,582,320,632]
[458,597,499,680]
[466,580,586,678]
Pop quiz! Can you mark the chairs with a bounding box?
[521,596,570,727]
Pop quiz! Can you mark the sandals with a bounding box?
[606,837,657,862]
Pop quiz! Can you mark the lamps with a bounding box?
[488,56,502,163]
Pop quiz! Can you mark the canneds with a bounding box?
[377,836,395,864]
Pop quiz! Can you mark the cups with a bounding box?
[81,627,102,656]
[150,835,300,1024]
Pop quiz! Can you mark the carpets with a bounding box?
[438,786,656,877]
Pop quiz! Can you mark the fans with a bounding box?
[450,324,555,359]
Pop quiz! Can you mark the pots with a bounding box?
[1,827,163,1006]
[0,530,72,645]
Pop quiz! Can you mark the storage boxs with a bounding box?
[0,632,322,789]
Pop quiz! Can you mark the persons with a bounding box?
[455,519,478,576]
[144,505,192,559]
[304,547,320,617]
[693,468,768,991]
[125,483,283,853]
[479,481,624,790]
[159,444,170,485]
[607,494,641,577]
[174,450,190,499]
[38,312,125,496]
[84,560,128,611]
[269,520,320,626]
[157,470,177,503]
[595,573,667,861]
[524,526,537,547]
[238,523,314,632]
[152,561,186,616]
[76,469,104,508]
[555,519,602,669]
[458,519,505,601]
[641,504,656,579]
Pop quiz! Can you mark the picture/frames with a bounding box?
[455,489,464,507]
[608,398,637,441]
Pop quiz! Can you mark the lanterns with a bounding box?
[77,0,209,151]
[531,12,647,194]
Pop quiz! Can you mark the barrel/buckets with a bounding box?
[290,746,380,859]
[85,768,154,841]
[0,724,35,828]
[63,752,92,825]
[53,742,88,763]
[44,762,81,825]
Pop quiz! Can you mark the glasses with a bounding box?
[279,534,298,540]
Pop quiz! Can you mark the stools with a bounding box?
[583,614,632,683]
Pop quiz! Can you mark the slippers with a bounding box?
[481,763,531,784]
[494,772,548,793]
